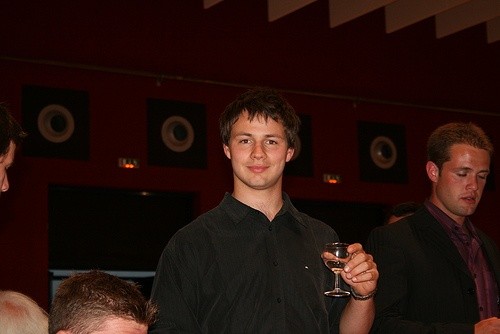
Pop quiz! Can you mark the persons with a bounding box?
[365,122,500,334]
[0,108,160,334]
[148,87,380,334]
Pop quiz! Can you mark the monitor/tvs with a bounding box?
[46,182,197,272]
[291,197,390,252]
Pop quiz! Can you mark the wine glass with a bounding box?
[323,243,350,296]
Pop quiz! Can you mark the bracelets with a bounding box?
[350,287,377,301]
